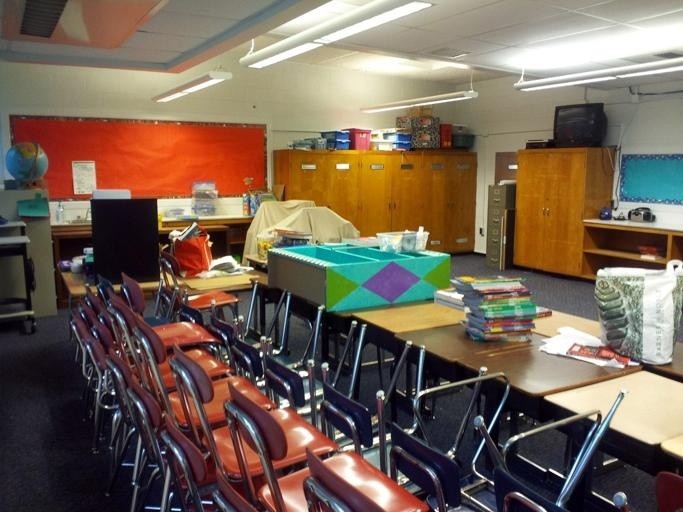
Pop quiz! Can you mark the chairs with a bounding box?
[212,469,263,510]
[65,249,683,512]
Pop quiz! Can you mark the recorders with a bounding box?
[627,207,655,222]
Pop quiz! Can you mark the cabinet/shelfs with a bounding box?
[513,146,616,278]
[580,218,683,281]
[485,182,517,272]
[272,148,477,253]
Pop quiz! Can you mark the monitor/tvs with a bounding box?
[553,103,608,147]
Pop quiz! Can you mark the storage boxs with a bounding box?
[303,138,327,150]
[340,128,372,151]
[265,240,452,314]
[326,139,351,150]
[382,133,412,142]
[319,131,350,140]
[369,140,392,151]
[391,141,411,151]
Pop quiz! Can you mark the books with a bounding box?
[191,180,218,216]
[166,208,199,221]
[242,190,279,216]
[435,276,552,348]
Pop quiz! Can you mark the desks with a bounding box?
[330,303,467,418]
[643,339,683,383]
[58,266,258,340]
[543,369,682,487]
[530,308,601,341]
[50,214,255,309]
[249,276,434,375]
[394,323,644,478]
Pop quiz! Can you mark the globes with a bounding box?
[5,141,51,190]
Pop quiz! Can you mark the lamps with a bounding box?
[513,55,683,93]
[239,1,438,71]
[150,53,233,103]
[358,69,479,115]
